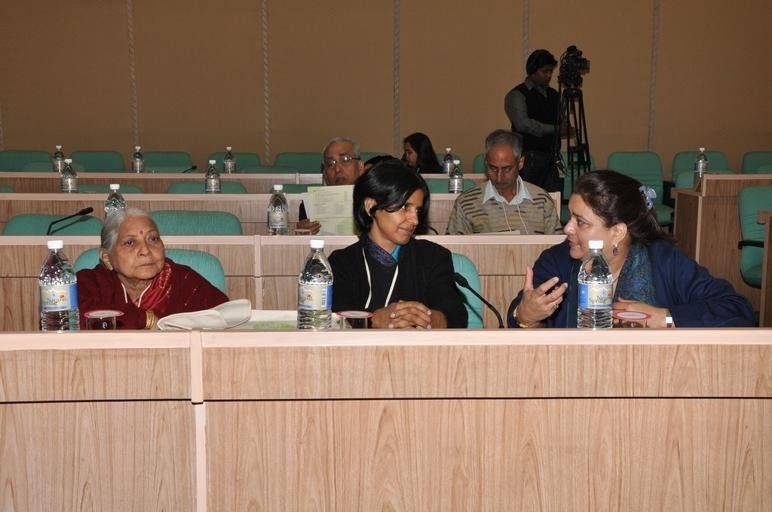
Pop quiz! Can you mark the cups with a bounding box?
[619,319,647,328]
[86,312,116,330]
[340,315,368,329]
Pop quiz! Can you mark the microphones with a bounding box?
[46,207,94,235]
[453,272,503,327]
[183,165,197,173]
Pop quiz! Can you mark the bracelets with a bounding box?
[146,305,156,331]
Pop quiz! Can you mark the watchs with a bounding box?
[665,307,674,328]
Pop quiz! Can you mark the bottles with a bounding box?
[448,160,463,194]
[576,238,613,328]
[204,160,221,194]
[265,184,290,235]
[693,147,708,189]
[38,239,82,334]
[222,146,237,172]
[442,147,452,172]
[132,146,145,174]
[53,145,78,193]
[294,237,333,332]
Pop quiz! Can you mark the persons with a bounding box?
[76,207,230,329]
[505,171,757,328]
[446,127,564,233]
[329,156,468,328]
[401,133,442,173]
[297,136,362,234]
[502,48,575,198]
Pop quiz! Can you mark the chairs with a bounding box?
[0,149,772,330]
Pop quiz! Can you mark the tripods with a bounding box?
[550,73,592,194]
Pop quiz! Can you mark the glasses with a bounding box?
[484,158,516,175]
[327,155,360,169]
